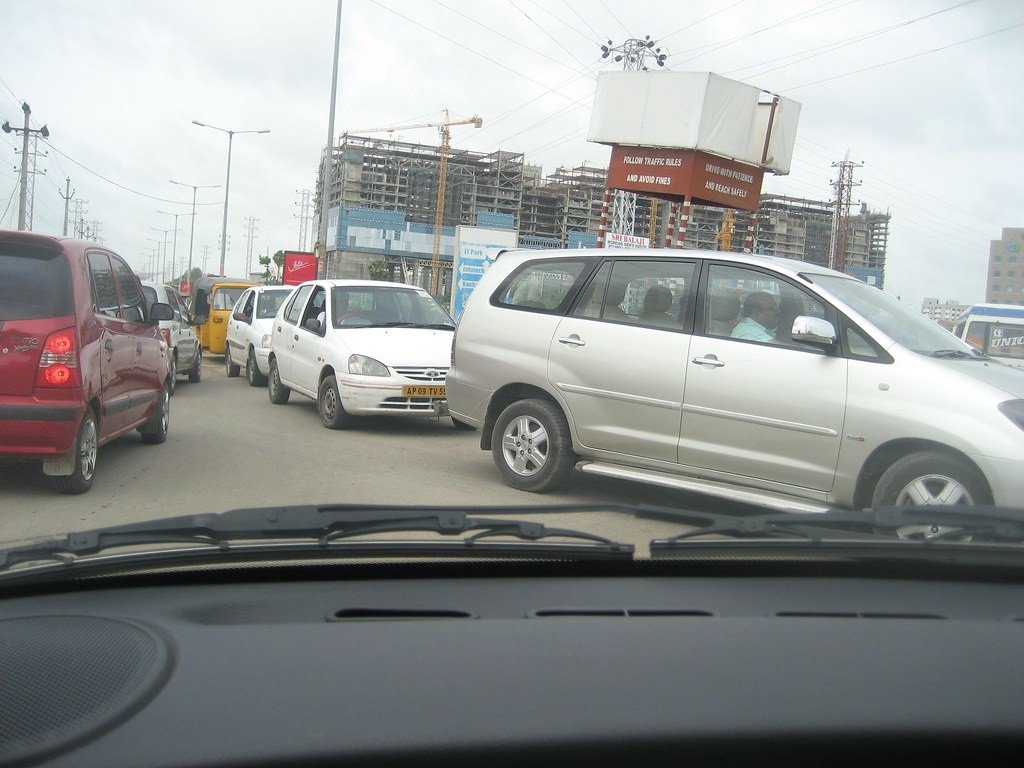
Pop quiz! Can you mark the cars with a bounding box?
[135,279,206,398]
[269,278,456,430]
[224,284,301,386]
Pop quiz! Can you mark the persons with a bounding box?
[729,290,787,346]
[316,292,361,328]
[255,296,277,316]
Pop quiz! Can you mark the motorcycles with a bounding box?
[189,277,256,355]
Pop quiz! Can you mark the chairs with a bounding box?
[639,284,683,330]
[308,295,326,320]
[590,274,631,322]
[709,288,742,337]
[776,300,809,347]
[375,295,400,324]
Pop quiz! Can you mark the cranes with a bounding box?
[341,106,484,298]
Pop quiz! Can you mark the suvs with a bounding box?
[443,245,1023,537]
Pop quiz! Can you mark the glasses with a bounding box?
[752,303,779,311]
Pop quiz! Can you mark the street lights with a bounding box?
[158,209,197,287]
[192,118,271,278]
[168,181,222,278]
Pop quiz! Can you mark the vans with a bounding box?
[1,229,177,496]
